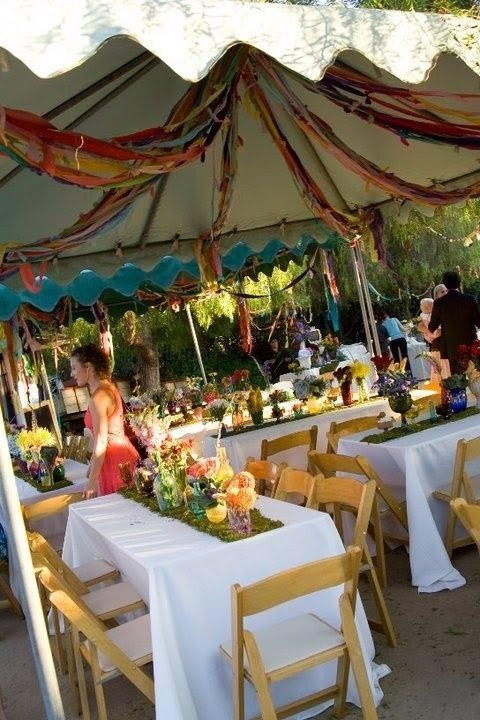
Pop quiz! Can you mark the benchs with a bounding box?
[67,493,335,720]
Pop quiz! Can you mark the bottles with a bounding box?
[429,401,439,425]
[440,388,453,420]
[186,482,206,521]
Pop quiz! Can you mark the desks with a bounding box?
[337,406,479,552]
[162,389,436,477]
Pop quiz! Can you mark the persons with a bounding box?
[260,338,294,383]
[428,272,480,393]
[378,309,413,377]
[424,284,448,380]
[418,298,441,337]
[70,345,142,499]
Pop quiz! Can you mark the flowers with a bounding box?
[6,418,67,485]
[119,399,253,529]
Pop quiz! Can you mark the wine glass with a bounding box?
[119,462,132,494]
[41,447,57,489]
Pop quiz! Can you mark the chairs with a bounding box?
[166,319,479,394]
[26,530,121,677]
[325,407,388,482]
[39,569,155,719]
[31,554,143,694]
[306,448,408,588]
[261,430,316,489]
[432,435,480,561]
[20,488,98,638]
[244,455,289,500]
[274,467,314,514]
[60,432,88,464]
[215,546,374,720]
[303,474,398,648]
[406,397,437,423]
